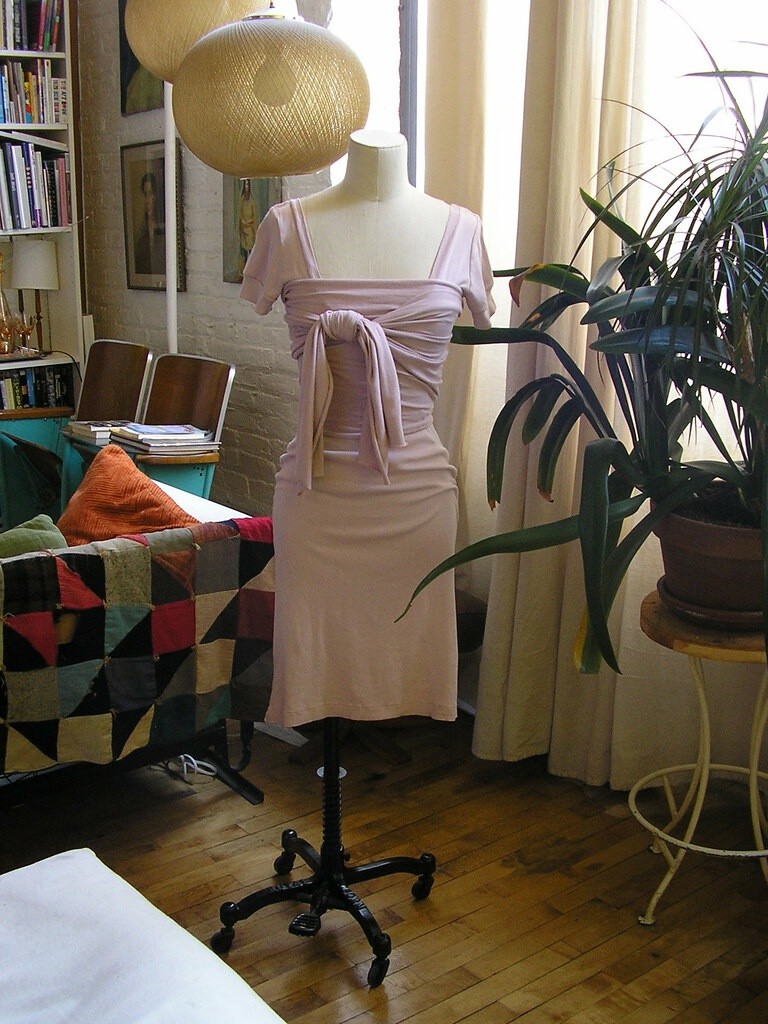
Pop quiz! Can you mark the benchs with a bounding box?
[0,478,278,804]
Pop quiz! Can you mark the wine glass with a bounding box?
[12,311,35,358]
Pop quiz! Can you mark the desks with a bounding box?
[0,847,287,1024]
[0,430,63,467]
[61,426,220,466]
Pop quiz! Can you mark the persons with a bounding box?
[238,128,497,728]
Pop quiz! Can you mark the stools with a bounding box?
[628,589,768,927]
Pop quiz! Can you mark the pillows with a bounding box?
[0,514,68,558]
[57,444,202,547]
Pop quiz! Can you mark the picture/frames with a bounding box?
[222,173,283,284]
[119,137,188,293]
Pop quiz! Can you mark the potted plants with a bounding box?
[393,0,768,674]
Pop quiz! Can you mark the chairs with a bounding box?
[10,339,153,518]
[80,353,237,500]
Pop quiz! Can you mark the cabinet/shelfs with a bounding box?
[0,0,89,420]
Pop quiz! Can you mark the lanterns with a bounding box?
[171,7,370,177]
[125,0,272,85]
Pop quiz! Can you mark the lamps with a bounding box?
[10,240,59,355]
[124,0,299,85]
[172,0,371,180]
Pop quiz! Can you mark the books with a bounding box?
[68,420,141,446]
[0,59,67,124]
[0,130,71,230]
[108,424,223,455]
[0,367,73,410]
[0,0,63,53]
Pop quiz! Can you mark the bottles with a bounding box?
[0,254,15,354]
